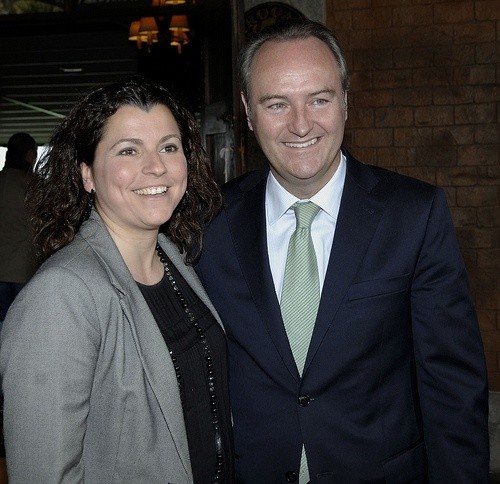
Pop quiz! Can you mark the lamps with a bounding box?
[128,0,197,55]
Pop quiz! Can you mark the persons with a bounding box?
[187,21,490,484]
[0,75,227,484]
[0,133,44,320]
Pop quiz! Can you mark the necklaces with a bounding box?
[136,244,223,484]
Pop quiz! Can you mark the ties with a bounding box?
[278,200,322,484]
